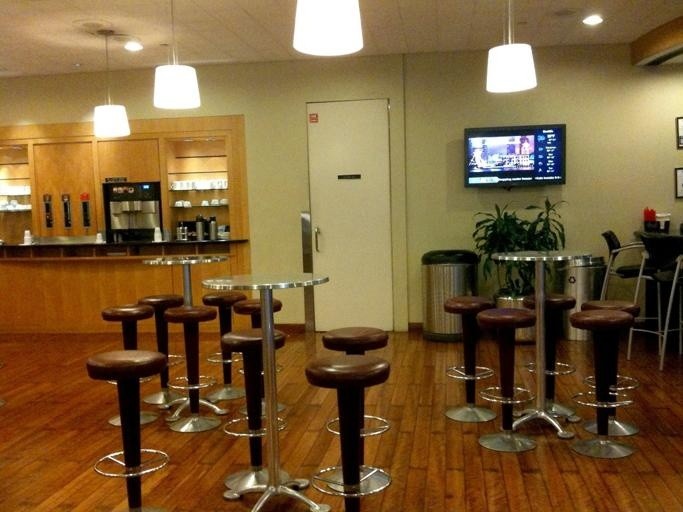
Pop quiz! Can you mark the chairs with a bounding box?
[627,231,683,370]
[600,230,649,300]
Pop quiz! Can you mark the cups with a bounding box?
[175,200,191,208]
[655,213,671,234]
[0,200,31,210]
[96,232,103,243]
[201,201,209,206]
[210,199,219,206]
[220,199,228,205]
[171,179,227,190]
[154,227,162,242]
[24,230,31,245]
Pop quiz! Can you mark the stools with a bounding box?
[322,327,392,496]
[102,305,159,428]
[306,355,392,512]
[582,301,642,437]
[235,298,287,416]
[570,310,636,460]
[524,294,576,418]
[165,306,222,434]
[202,292,247,401]
[86,350,171,512]
[476,309,538,454]
[222,328,289,493]
[444,296,498,422]
[139,294,185,405]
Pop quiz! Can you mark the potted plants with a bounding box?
[472,196,568,343]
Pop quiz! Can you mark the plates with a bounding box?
[152,240,167,243]
[18,244,34,245]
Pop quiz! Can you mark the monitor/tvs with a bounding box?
[463,124,567,188]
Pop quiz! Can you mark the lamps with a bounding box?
[92,31,132,139]
[154,2,202,110]
[485,0,539,92]
[293,0,364,57]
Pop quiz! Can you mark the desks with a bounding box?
[490,250,581,440]
[203,275,332,512]
[142,256,232,422]
